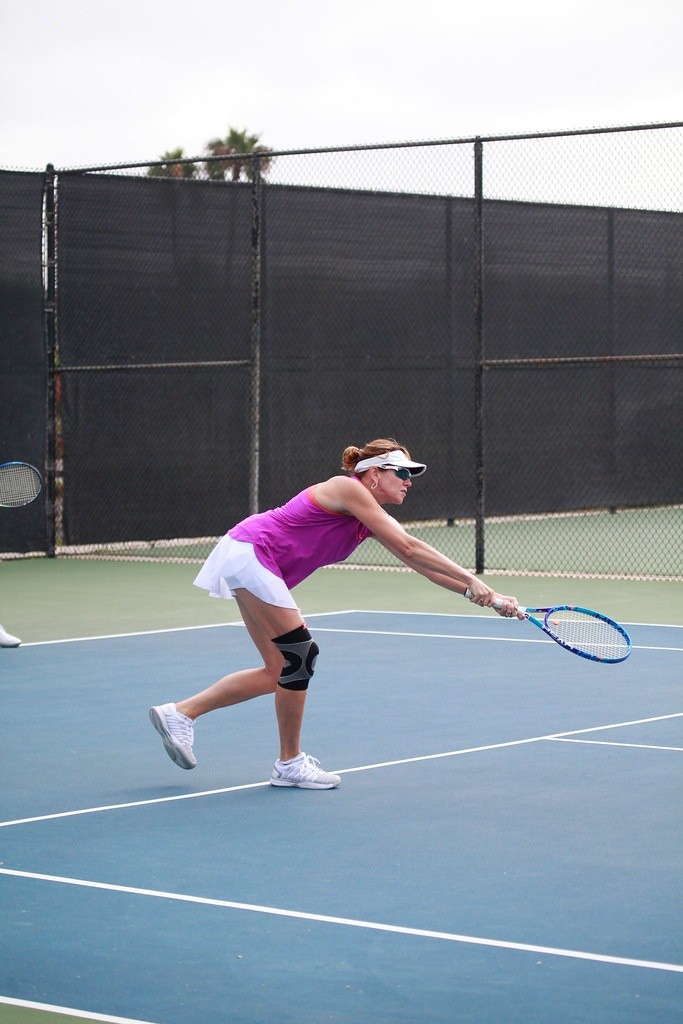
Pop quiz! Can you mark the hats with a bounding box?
[353,450,427,478]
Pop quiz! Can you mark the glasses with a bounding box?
[377,463,411,482]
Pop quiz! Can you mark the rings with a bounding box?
[480,599,484,604]
[506,612,511,616]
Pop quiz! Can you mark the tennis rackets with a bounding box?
[464,587,632,664]
[0,461,44,508]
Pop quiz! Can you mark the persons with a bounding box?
[147,439,527,789]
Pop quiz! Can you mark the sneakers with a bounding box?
[0,625,21,647]
[270,752,341,790]
[149,703,197,770]
